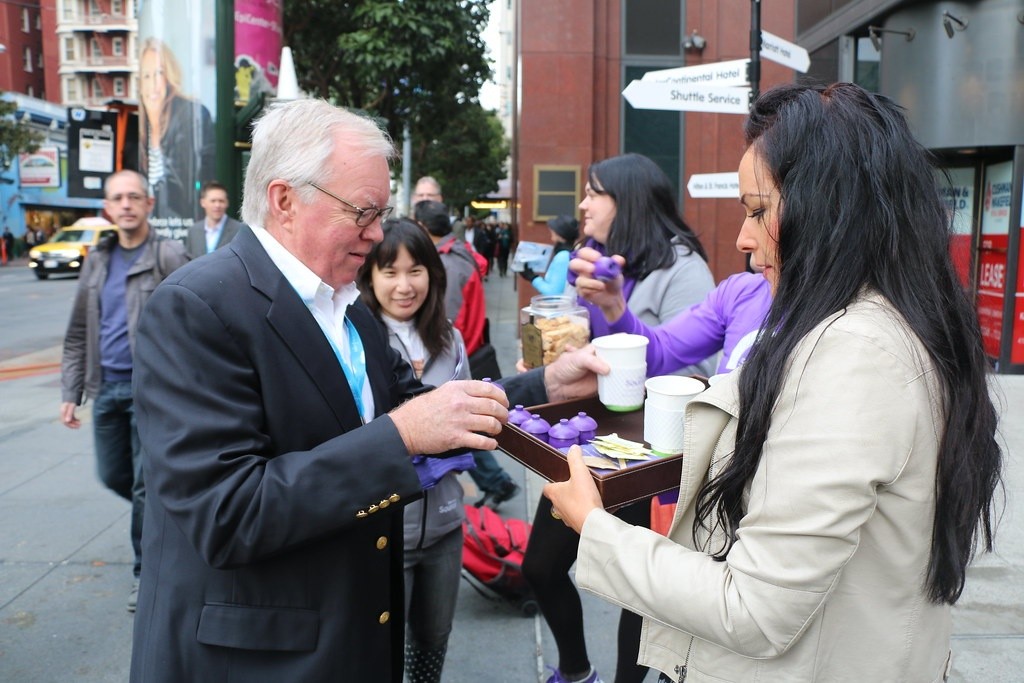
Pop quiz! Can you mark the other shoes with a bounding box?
[126,576,140,611]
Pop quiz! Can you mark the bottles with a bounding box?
[519,293,590,371]
[479,375,597,448]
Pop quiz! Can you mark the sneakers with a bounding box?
[474,477,522,512]
[546,650,605,683]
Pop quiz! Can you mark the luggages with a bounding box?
[461,502,538,617]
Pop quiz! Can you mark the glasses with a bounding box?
[414,192,442,199]
[305,179,394,227]
[104,192,148,203]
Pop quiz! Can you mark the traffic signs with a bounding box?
[620,30,812,198]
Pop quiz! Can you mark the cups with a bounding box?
[591,333,649,413]
[644,374,705,457]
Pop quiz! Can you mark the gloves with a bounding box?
[519,264,539,283]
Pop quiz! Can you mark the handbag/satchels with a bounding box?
[468,345,502,383]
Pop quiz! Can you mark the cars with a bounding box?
[28,217,121,280]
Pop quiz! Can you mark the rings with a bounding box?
[550,507,561,520]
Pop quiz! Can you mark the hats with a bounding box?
[547,213,580,240]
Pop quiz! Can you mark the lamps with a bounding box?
[868,26,916,51]
[943,10,970,39]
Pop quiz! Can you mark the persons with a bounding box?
[411,176,514,280]
[543,81,1007,683]
[515,152,723,683]
[129,100,611,683]
[59,170,193,613]
[570,247,784,378]
[520,214,581,295]
[355,217,473,683]
[411,199,522,511]
[131,37,212,245]
[3,222,56,261]
[185,181,242,261]
